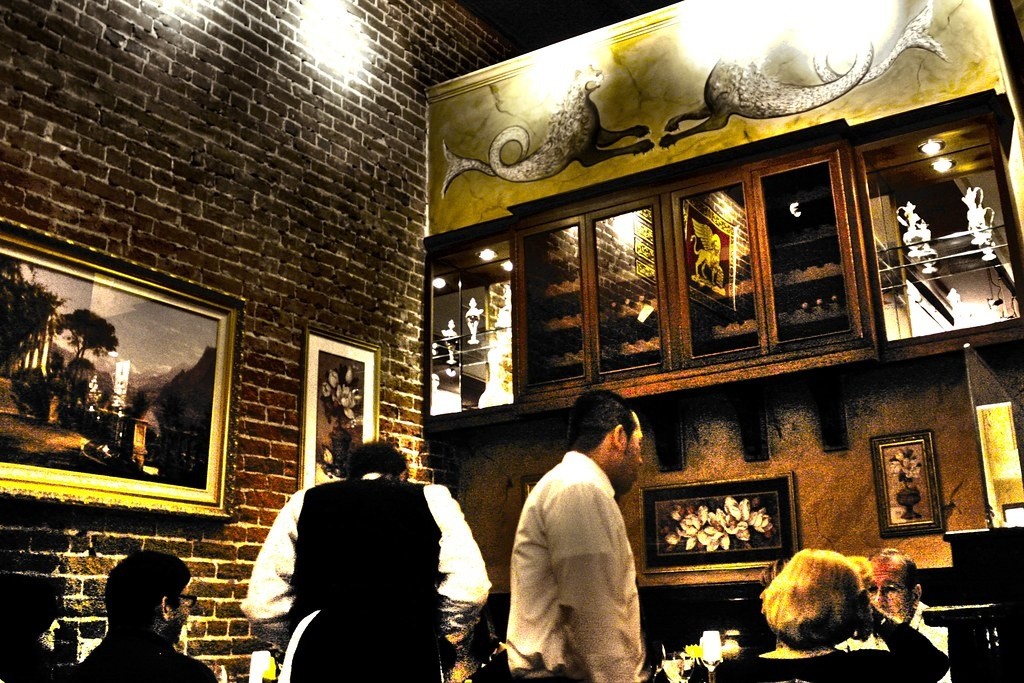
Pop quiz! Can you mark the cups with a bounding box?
[662,652,694,683]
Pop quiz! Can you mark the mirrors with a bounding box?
[845,121,1024,346]
[421,225,519,422]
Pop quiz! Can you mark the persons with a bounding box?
[54,549,219,683]
[714,548,952,683]
[240,440,492,683]
[506,389,643,683]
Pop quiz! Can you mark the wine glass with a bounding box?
[700,631,722,683]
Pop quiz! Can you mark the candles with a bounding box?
[721,642,742,658]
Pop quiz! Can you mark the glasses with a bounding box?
[177,593,197,608]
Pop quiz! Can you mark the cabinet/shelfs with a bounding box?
[512,180,677,405]
[658,136,875,371]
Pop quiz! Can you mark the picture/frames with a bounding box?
[519,471,546,507]
[0,213,247,526]
[635,469,801,575]
[296,321,383,498]
[868,427,947,539]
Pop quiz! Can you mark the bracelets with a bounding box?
[873,616,888,639]
